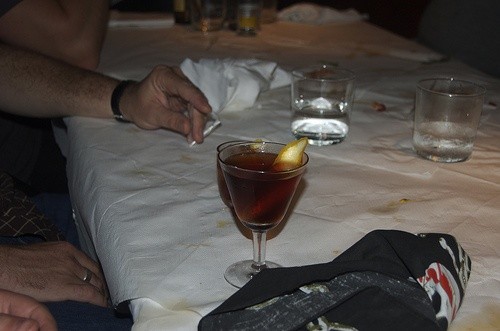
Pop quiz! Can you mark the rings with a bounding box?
[80,269,95,282]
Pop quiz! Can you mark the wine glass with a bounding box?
[218,141,309,289]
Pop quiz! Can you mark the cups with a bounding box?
[413,78,487,163]
[174,0,261,36]
[290,64,355,147]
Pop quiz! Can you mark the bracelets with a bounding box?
[109,77,141,122]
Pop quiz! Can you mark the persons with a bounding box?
[0,287,58,331]
[0,40,216,330]
[0,0,113,239]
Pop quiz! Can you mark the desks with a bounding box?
[53,12,500,331]
